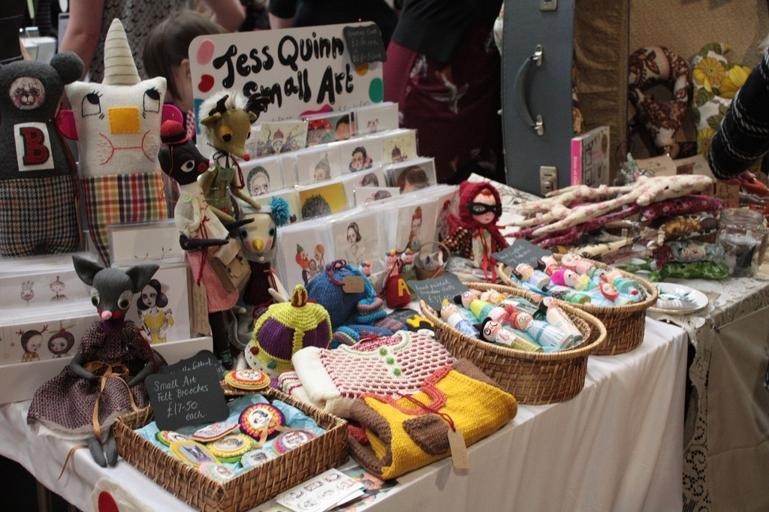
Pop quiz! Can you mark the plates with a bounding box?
[645,282,710,314]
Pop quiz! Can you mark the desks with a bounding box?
[17,300,689,512]
[611,242,769,512]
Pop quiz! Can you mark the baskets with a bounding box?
[111,382,351,511]
[498,249,659,355]
[418,281,611,405]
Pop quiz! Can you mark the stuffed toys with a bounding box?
[438,179,507,266]
[0,50,84,259]
[63,17,171,264]
[232,198,289,335]
[158,119,257,368]
[25,254,165,469]
[436,248,644,355]
[198,90,263,316]
